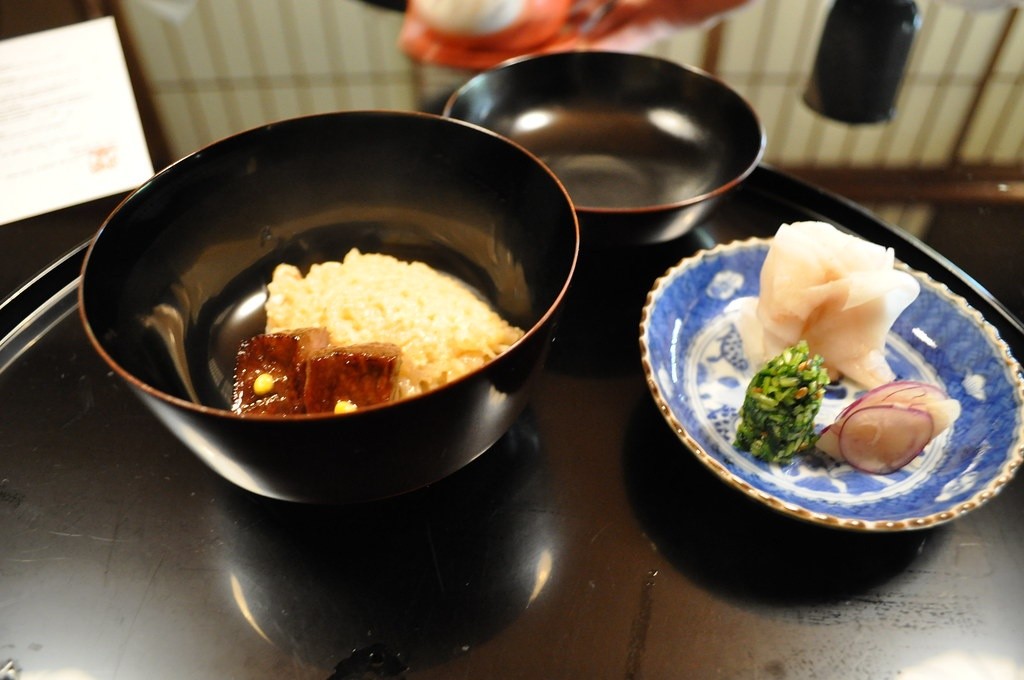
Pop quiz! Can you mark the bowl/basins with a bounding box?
[642,236,1024,533]
[443,46,767,253]
[79,110,580,506]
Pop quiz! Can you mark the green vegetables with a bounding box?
[734,338,832,466]
